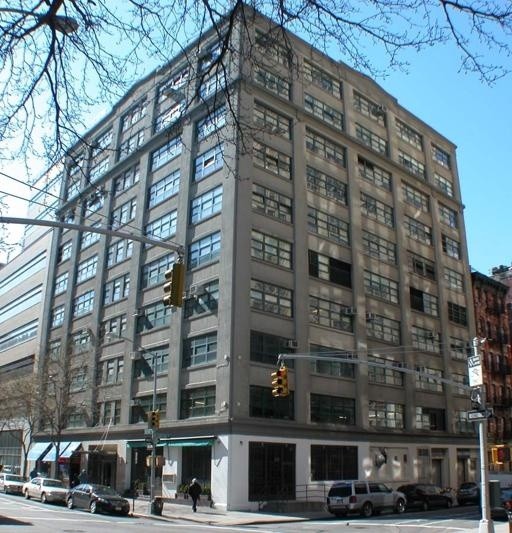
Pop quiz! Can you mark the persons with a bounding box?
[71,472,80,487]
[187,477,202,512]
[30,467,38,480]
[502,500,512,533]
[78,468,89,484]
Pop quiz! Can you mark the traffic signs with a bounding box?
[467,408,495,421]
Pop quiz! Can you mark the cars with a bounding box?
[326,479,408,517]
[456,482,481,505]
[22,477,70,503]
[396,482,454,511]
[64,484,130,515]
[502,487,512,512]
[0,473,27,494]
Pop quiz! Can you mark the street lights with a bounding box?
[105,331,158,503]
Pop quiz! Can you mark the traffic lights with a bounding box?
[146,410,160,430]
[163,263,184,308]
[487,444,511,465]
[271,367,289,398]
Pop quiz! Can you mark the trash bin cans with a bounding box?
[489,480,501,506]
[152,498,164,515]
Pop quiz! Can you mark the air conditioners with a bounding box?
[130,399,139,406]
[366,312,376,319]
[190,283,204,295]
[346,307,358,314]
[130,352,141,360]
[284,339,299,348]
[428,331,438,337]
